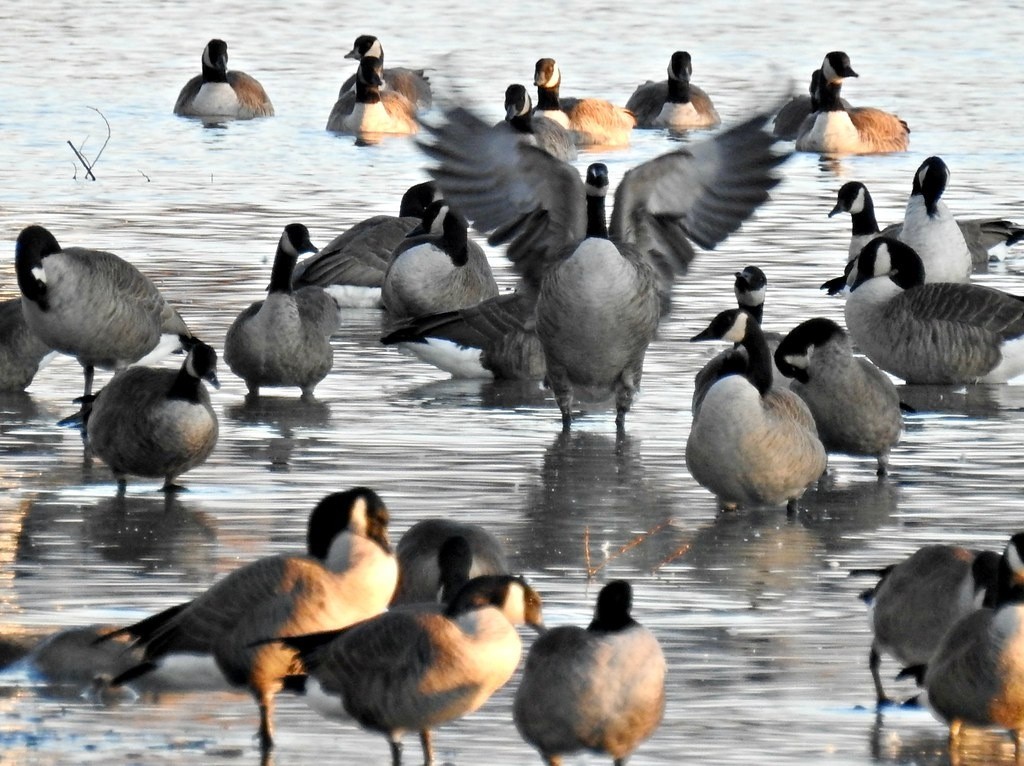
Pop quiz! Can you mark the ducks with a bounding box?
[0,298,54,392]
[73,344,222,496]
[326,35,432,134]
[172,40,274,120]
[90,480,400,766]
[293,50,1021,517]
[511,579,666,766]
[845,533,1024,765]
[393,517,509,607]
[14,226,193,425]
[222,223,342,400]
[243,576,548,766]
